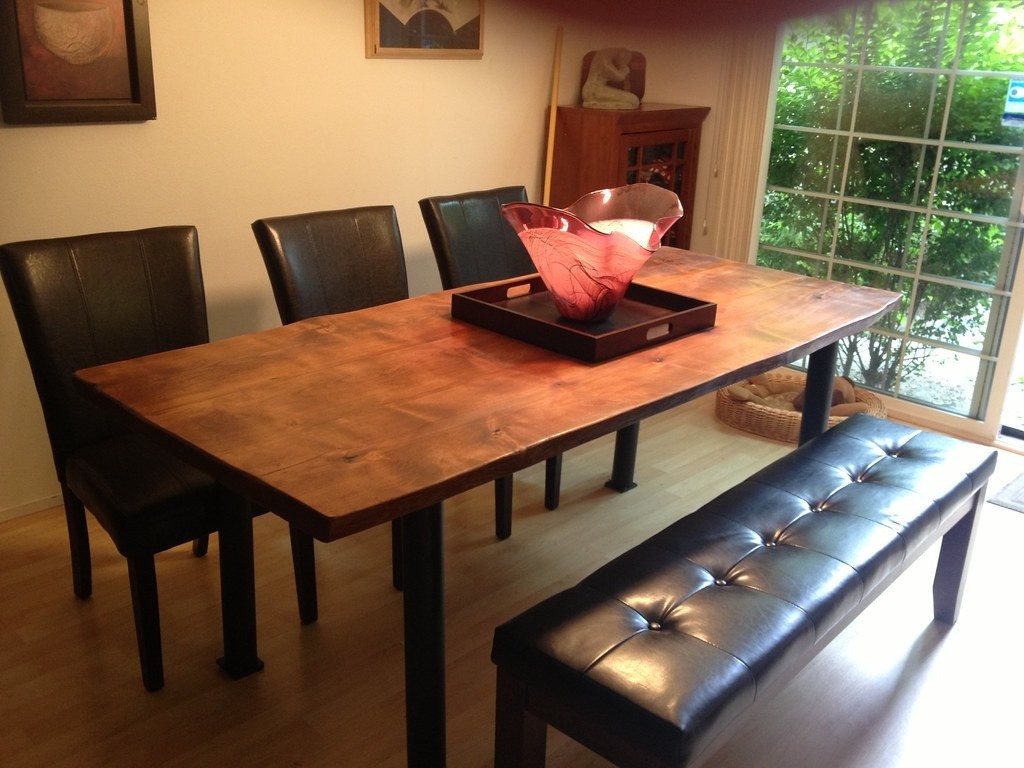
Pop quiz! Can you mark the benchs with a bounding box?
[490,411,999,768]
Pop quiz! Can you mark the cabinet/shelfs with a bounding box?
[544,100,711,249]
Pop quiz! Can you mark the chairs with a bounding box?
[419,186,563,509]
[251,203,512,587]
[0,225,317,692]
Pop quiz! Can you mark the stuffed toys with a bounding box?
[725,376,869,416]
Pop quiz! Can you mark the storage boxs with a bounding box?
[452,272,719,366]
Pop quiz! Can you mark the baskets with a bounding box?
[714,372,887,443]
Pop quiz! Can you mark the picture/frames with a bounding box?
[363,0,486,60]
[0,0,157,126]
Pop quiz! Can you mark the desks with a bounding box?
[77,247,904,768]
